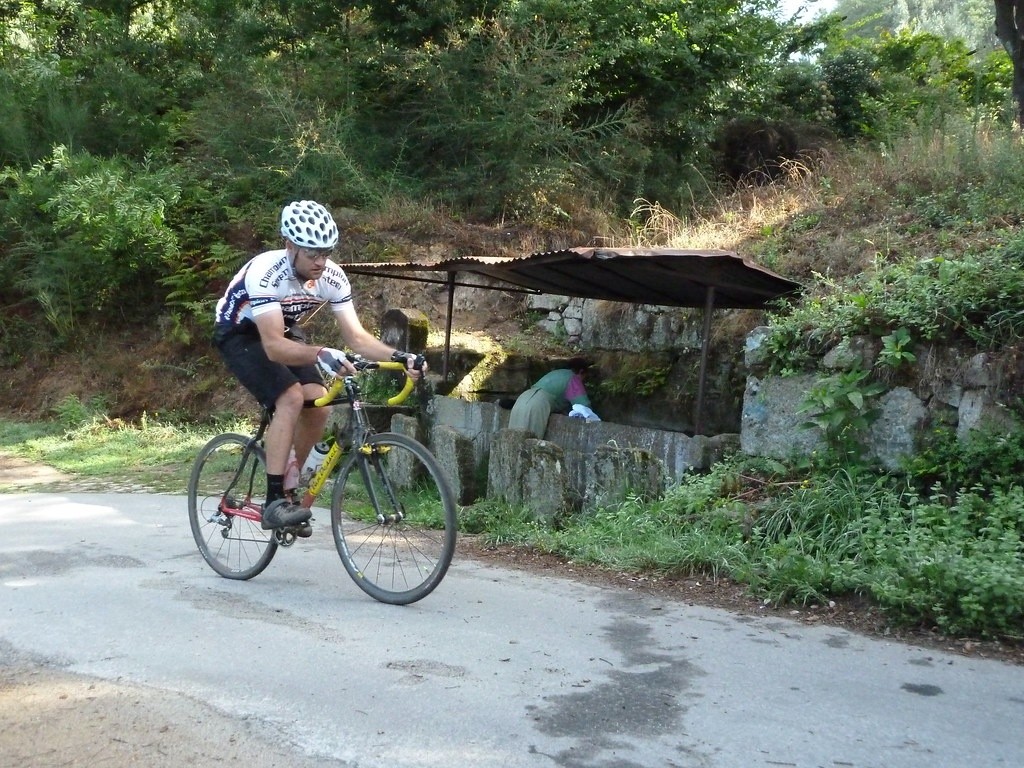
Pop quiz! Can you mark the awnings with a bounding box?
[333,245,815,309]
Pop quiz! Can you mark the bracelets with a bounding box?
[390,350,401,360]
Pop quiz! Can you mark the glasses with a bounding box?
[302,249,332,257]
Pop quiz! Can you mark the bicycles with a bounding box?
[188,352,458,606]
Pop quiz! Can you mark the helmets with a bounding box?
[280,200,339,248]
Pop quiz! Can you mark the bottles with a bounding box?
[298,442,329,488]
[283,444,298,492]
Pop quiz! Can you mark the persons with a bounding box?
[509,356,592,440]
[207,200,429,542]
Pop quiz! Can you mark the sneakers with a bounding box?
[261,499,312,530]
[289,501,312,537]
